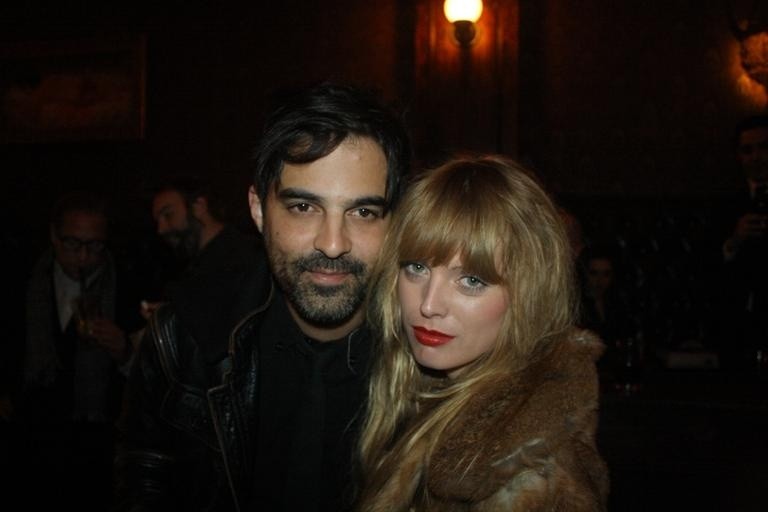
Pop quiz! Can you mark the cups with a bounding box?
[611,338,640,369]
[71,294,102,339]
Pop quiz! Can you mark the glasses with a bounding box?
[59,235,103,252]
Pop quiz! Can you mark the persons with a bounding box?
[143,182,264,366]
[353,152,609,511]
[698,112,768,394]
[2,196,165,422]
[586,253,623,325]
[119,90,412,510]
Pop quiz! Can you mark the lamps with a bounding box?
[444,0,483,49]
